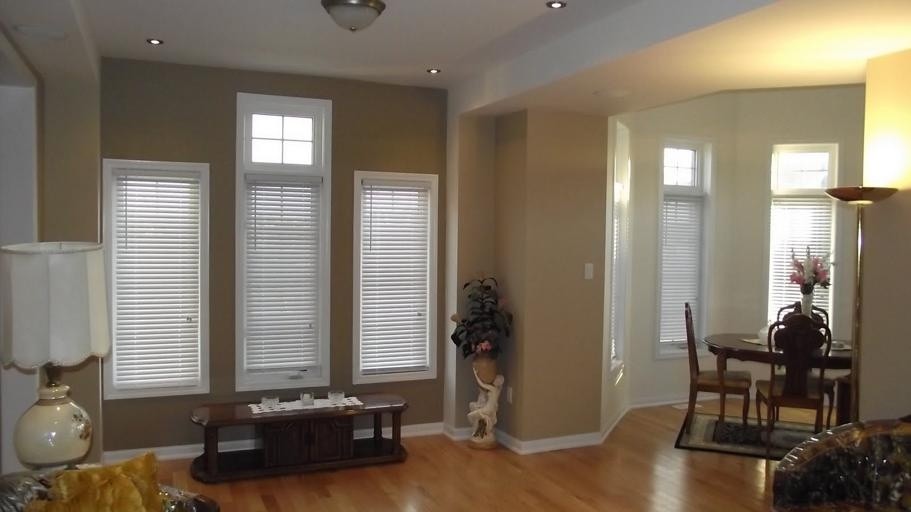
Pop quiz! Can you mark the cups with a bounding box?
[327,391,345,405]
[299,391,316,406]
[260,395,280,411]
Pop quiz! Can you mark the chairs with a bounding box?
[681,302,752,434]
[0,463,220,512]
[755,300,838,459]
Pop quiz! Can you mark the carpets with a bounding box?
[674,412,816,462]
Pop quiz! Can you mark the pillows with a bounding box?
[25,451,169,512]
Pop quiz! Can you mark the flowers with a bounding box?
[450,275,513,359]
[786,243,839,295]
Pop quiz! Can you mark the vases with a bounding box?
[800,294,814,319]
[475,353,496,384]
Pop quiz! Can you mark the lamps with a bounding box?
[321,0,385,32]
[823,185,899,422]
[0,241,111,472]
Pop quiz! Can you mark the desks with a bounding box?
[190,392,410,485]
